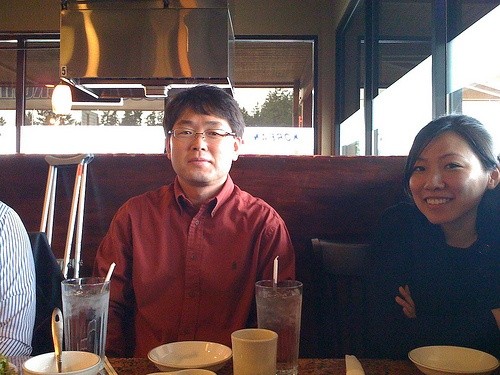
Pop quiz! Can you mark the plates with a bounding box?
[149,367,218,375]
[8,361,18,375]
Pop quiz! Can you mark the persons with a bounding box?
[0,201,36,357]
[362,114,500,360]
[85,85,296,357]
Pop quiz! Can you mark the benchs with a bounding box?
[0,153,409,357]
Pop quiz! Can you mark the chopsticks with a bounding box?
[103,356,118,375]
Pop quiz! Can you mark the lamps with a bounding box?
[51,81,72,115]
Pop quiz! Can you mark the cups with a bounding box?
[61,275,110,362]
[254,279,303,375]
[231,328,279,375]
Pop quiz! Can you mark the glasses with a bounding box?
[168,128,236,144]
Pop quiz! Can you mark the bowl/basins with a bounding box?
[22,350,102,375]
[146,339,232,372]
[408,345,500,375]
